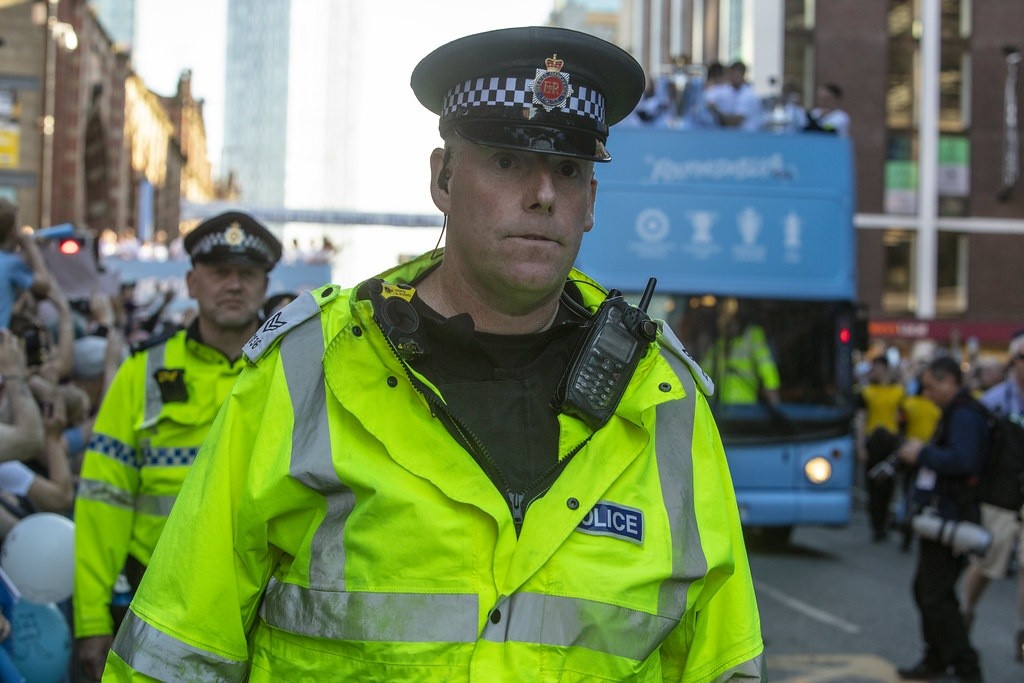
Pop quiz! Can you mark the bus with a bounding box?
[574,124,869,551]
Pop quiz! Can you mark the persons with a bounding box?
[690,303,780,409]
[96,30,767,683]
[0,190,295,683]
[852,328,1024,683]
[69,211,283,683]
[631,51,848,137]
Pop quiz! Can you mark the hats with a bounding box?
[183,212,282,273]
[411,26,645,163]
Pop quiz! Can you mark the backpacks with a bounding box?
[940,399,1024,512]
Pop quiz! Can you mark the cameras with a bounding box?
[869,453,903,485]
[910,512,995,558]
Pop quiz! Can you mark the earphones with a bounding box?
[437,168,451,194]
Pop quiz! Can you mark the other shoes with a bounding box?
[897,656,946,680]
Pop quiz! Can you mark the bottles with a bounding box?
[111,575,131,607]
